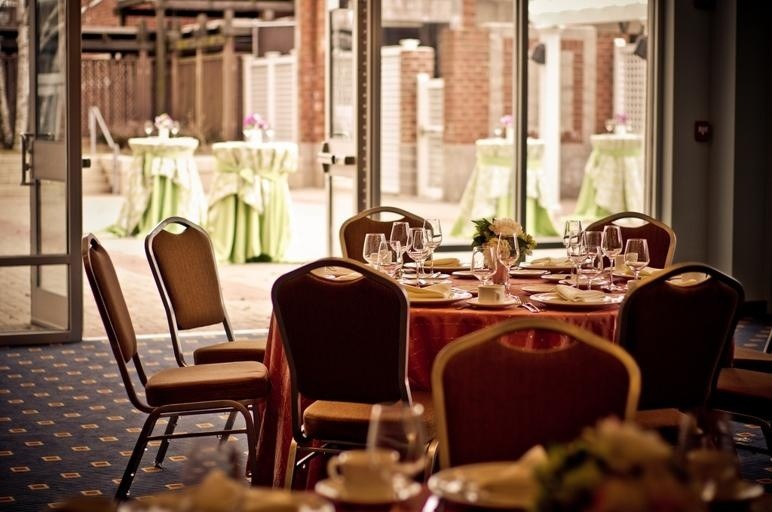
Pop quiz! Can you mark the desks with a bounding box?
[108,135,209,243]
[568,134,647,228]
[210,141,307,267]
[450,138,562,242]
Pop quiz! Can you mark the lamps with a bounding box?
[613,27,645,64]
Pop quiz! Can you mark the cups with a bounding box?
[477,283,506,305]
[327,449,398,501]
[180,436,247,512]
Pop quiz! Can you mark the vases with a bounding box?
[613,125,629,135]
[159,127,169,142]
[249,129,264,146]
[505,126,516,139]
[479,255,510,287]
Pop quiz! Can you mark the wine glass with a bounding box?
[353,215,520,295]
[555,218,650,291]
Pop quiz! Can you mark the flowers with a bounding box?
[238,114,270,129]
[469,219,535,264]
[608,110,633,123]
[498,115,516,122]
[155,114,176,125]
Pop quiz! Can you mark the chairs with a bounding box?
[337,200,438,268]
[424,318,645,476]
[140,213,272,453]
[75,233,278,501]
[267,253,432,487]
[608,252,746,449]
[714,357,771,452]
[574,212,676,274]
[730,320,770,370]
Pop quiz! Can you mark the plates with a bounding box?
[469,297,523,310]
[117,487,333,511]
[537,272,569,282]
[525,291,622,310]
[427,460,547,508]
[506,270,548,279]
[308,476,422,504]
[408,288,471,305]
